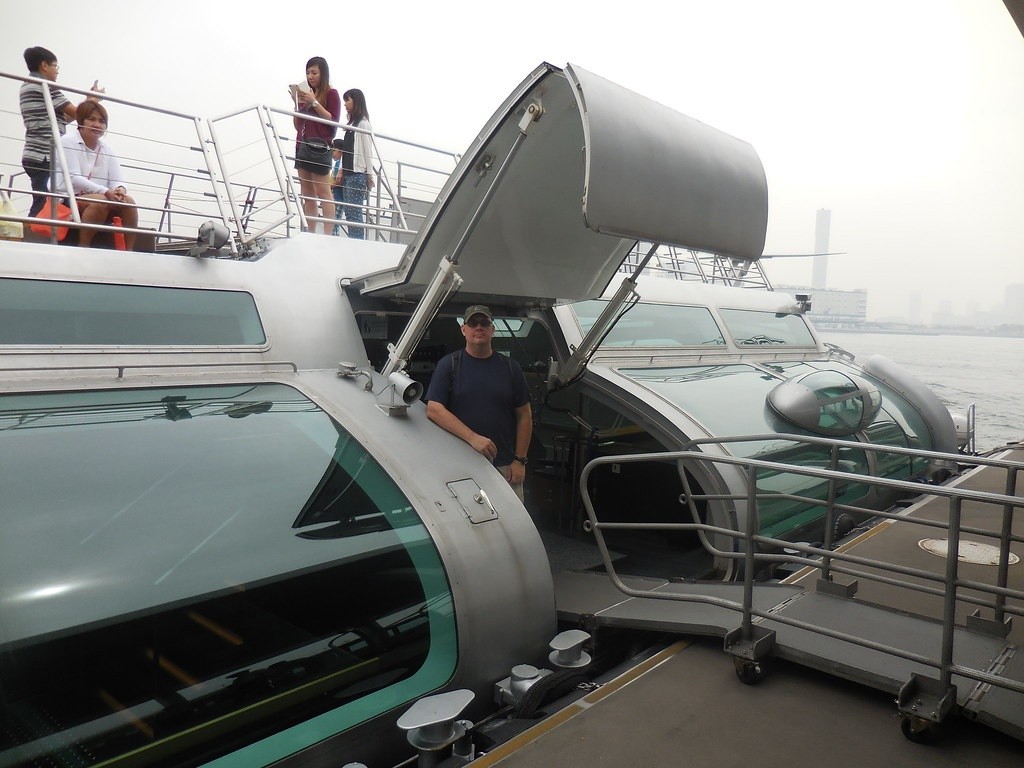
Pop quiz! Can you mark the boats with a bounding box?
[0,60,979,768]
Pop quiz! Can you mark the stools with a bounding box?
[58,216,115,250]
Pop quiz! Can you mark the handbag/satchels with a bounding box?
[294,136,333,176]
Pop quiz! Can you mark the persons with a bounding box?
[19,45,108,220]
[289,56,341,236]
[423,305,532,511]
[340,89,376,242]
[326,139,346,237]
[43,101,138,251]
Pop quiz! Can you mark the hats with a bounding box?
[464,305,494,325]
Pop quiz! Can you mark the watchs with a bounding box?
[513,455,528,464]
[312,100,318,108]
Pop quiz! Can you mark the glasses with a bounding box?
[466,319,492,328]
[45,62,59,70]
